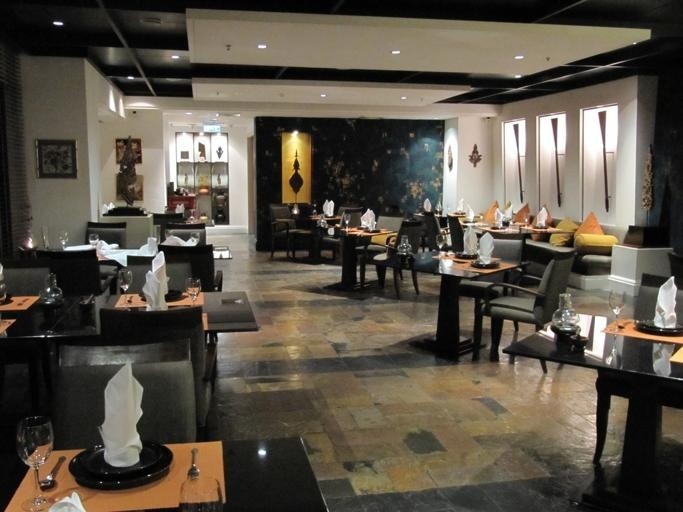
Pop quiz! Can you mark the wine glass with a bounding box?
[609,289,626,333]
[88,233,100,249]
[604,335,621,369]
[56,231,73,250]
[15,416,56,508]
[185,278,202,307]
[435,234,446,259]
[343,214,352,233]
[118,268,133,306]
[151,225,161,244]
[190,232,200,246]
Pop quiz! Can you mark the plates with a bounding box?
[69,440,173,492]
[637,319,683,335]
[471,259,500,269]
[139,287,182,301]
[454,251,479,258]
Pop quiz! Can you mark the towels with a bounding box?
[180,204,184,213]
[96,240,127,267]
[464,226,477,255]
[161,236,198,245]
[369,210,375,230]
[100,362,143,467]
[654,276,677,329]
[466,205,474,221]
[323,200,329,216]
[505,205,512,219]
[176,204,180,213]
[138,238,156,256]
[457,198,463,212]
[537,207,547,226]
[329,200,334,216]
[48,492,86,511]
[495,208,502,227]
[480,232,494,264]
[424,199,431,212]
[360,208,370,227]
[152,251,168,294]
[293,205,299,214]
[109,202,115,210]
[103,204,109,214]
[143,271,168,311]
[0,264,3,280]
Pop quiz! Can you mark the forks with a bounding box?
[16,298,30,306]
[187,449,200,479]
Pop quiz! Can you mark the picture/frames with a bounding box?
[34,138,78,179]
[114,137,141,164]
[115,173,144,203]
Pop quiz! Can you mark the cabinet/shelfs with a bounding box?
[174,132,228,225]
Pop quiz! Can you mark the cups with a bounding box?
[179,478,223,500]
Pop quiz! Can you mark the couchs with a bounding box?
[479,206,624,291]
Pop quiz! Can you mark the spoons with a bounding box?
[127,296,133,304]
[38,456,66,489]
[617,320,633,329]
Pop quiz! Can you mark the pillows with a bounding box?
[483,201,498,222]
[549,216,578,247]
[530,205,553,228]
[573,211,604,239]
[514,203,530,225]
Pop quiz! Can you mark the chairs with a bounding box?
[160,222,205,244]
[446,213,464,252]
[1,269,51,292]
[360,221,424,299]
[480,253,576,374]
[316,206,364,263]
[38,339,199,449]
[423,212,451,252]
[98,306,216,392]
[125,255,210,293]
[269,205,310,260]
[1,248,118,295]
[157,245,222,293]
[84,221,126,244]
